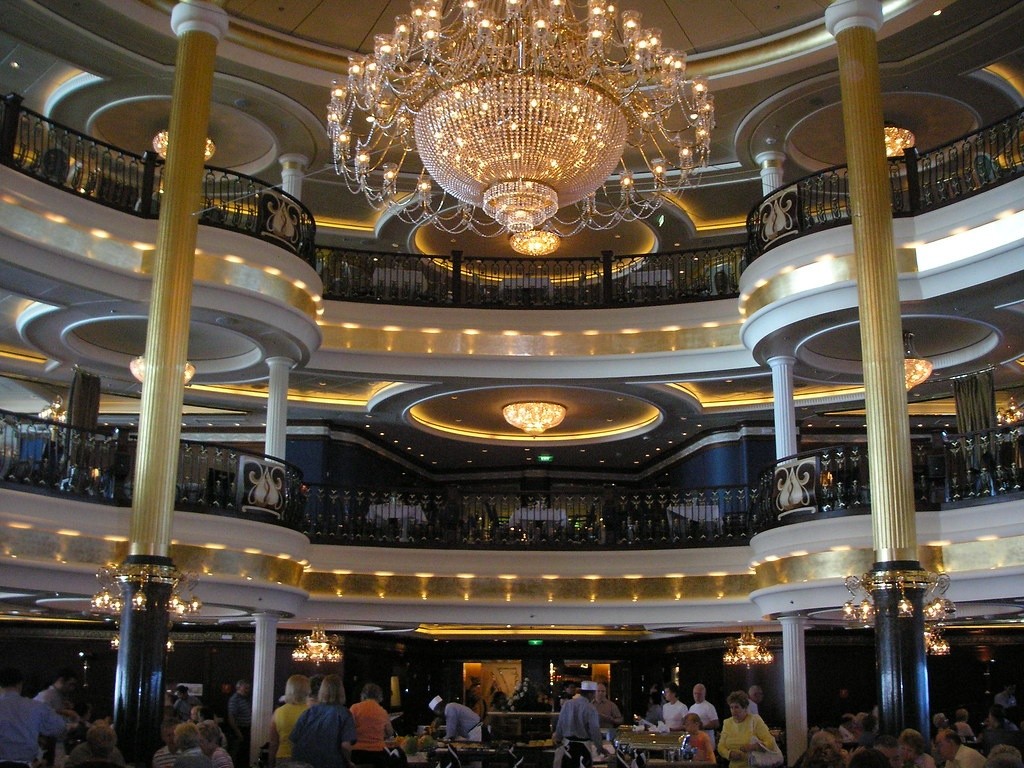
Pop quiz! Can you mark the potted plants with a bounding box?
[418,735,434,762]
[402,736,419,762]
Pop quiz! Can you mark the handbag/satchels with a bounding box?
[747,714,783,768]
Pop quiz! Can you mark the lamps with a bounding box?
[884,123,915,157]
[722,627,775,665]
[326,0,717,243]
[130,351,194,383]
[509,231,560,256]
[503,402,566,434]
[89,567,203,652]
[290,624,344,663]
[842,572,951,655]
[153,129,215,161]
[902,333,933,391]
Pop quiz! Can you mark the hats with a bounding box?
[581,681,598,690]
[429,695,442,710]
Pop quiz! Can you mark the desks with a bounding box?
[370,504,423,543]
[514,508,568,536]
[372,269,425,302]
[501,277,552,307]
[667,504,719,539]
[627,269,673,303]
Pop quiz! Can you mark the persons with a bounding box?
[152,677,234,768]
[746,685,764,714]
[793,703,1024,768]
[555,680,610,768]
[428,694,489,768]
[718,690,776,768]
[662,682,688,731]
[268,673,357,768]
[465,671,624,741]
[993,681,1016,709]
[348,683,395,768]
[0,667,125,768]
[228,680,252,768]
[688,683,719,752]
[682,713,716,764]
